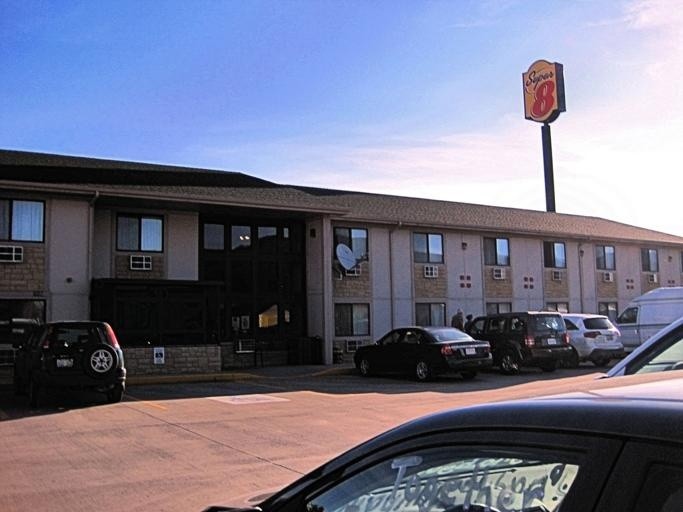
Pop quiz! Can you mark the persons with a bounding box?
[463,314,477,334]
[450,307,464,331]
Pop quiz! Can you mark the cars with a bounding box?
[353,325,494,382]
[597,317,683,379]
[201,370,682,512]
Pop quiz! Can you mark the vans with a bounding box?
[611,287,683,353]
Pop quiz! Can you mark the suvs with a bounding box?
[559,313,624,369]
[12,320,127,409]
[462,310,573,376]
